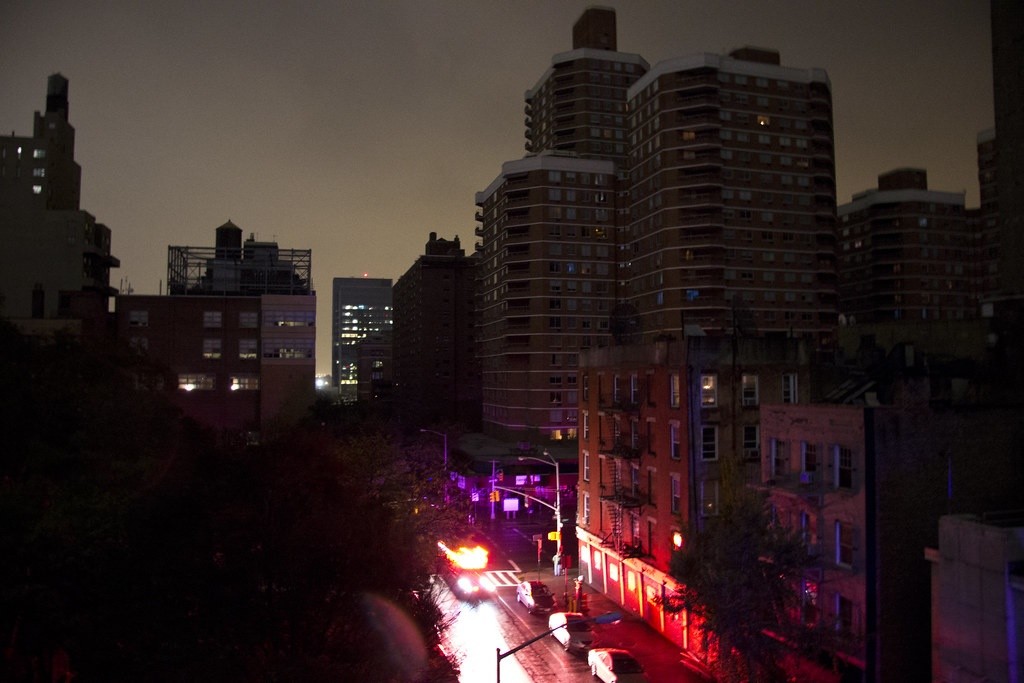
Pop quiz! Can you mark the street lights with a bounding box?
[496,611,623,683]
[419,429,447,471]
[518,451,561,554]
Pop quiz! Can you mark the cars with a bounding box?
[549,612,601,653]
[516,580,559,615]
[587,648,644,683]
